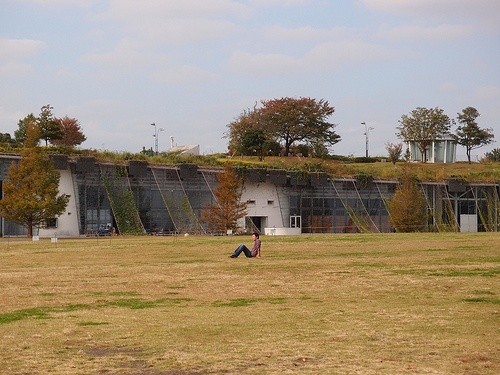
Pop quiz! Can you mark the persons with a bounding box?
[228,233,262,258]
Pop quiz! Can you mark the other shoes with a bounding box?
[230,255,238,258]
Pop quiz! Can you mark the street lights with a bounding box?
[361,121,375,157]
[151,123,164,155]
